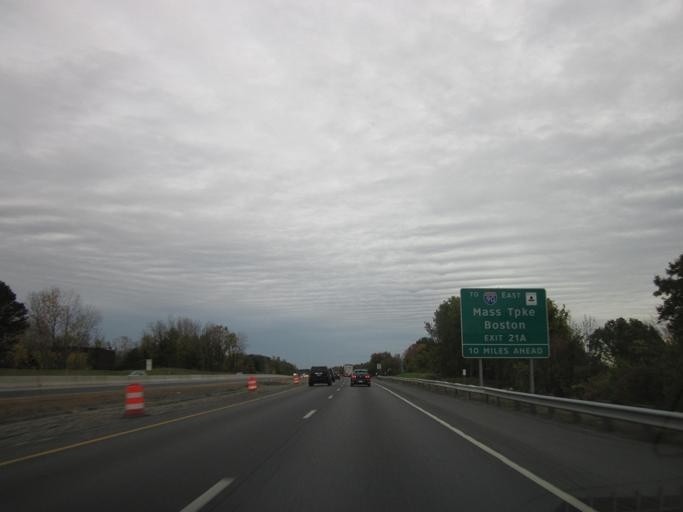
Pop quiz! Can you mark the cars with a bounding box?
[128,370,144,376]
[308,366,371,386]
